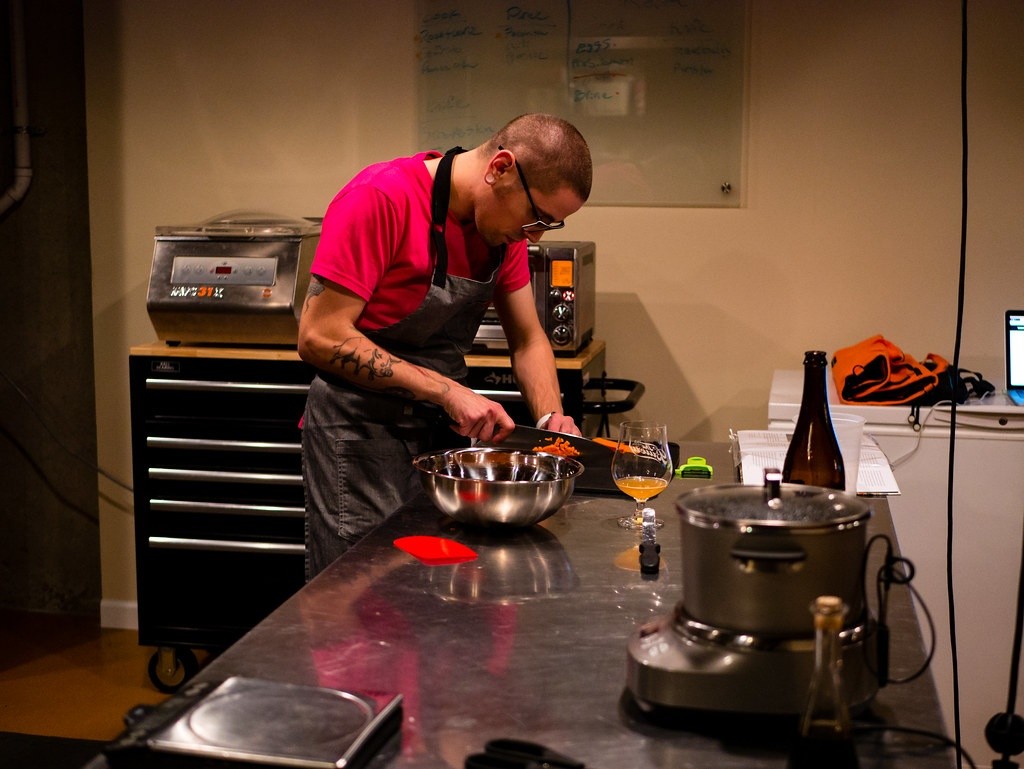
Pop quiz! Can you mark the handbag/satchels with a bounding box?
[831,333,948,406]
[898,353,995,405]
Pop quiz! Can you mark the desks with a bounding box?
[90,478,960,769]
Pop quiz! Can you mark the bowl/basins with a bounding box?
[413,447,584,524]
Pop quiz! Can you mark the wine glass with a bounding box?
[612,421,673,531]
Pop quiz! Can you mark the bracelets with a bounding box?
[535,413,552,430]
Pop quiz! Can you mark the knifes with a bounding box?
[418,402,614,467]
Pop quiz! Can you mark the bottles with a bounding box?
[781,350,845,491]
[783,597,853,769]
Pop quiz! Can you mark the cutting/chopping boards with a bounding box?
[496,435,680,499]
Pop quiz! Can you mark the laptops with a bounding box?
[1004,310,1024,406]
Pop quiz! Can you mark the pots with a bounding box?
[679,472,873,636]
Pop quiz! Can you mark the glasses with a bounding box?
[499,145,564,231]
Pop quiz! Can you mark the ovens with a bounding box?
[473,242,597,358]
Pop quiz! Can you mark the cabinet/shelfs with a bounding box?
[122,343,608,690]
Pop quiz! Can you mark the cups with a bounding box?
[793,414,866,498]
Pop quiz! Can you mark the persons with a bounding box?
[296,110,592,585]
